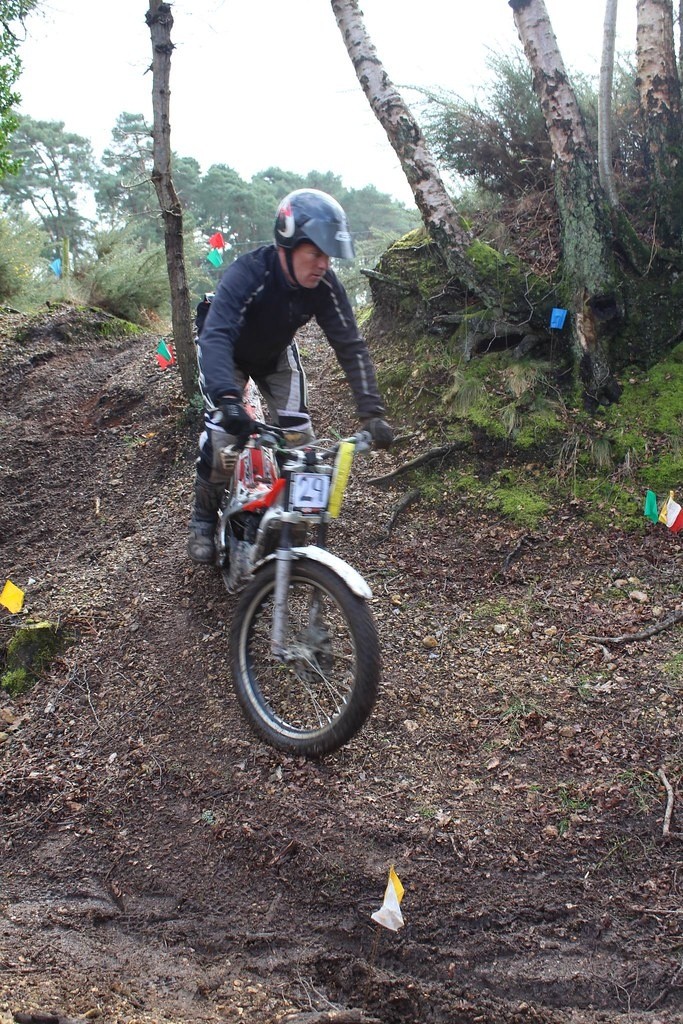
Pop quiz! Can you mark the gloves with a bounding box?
[219,396,257,451]
[362,415,393,451]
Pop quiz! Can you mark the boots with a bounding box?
[186,472,226,565]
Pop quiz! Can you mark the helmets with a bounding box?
[274,188,356,259]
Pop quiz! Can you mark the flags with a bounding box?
[0,580,23,613]
[48,257,61,274]
[205,248,223,268]
[370,864,405,933]
[155,339,176,371]
[643,489,658,524]
[658,496,683,532]
[550,308,567,330]
[208,232,225,248]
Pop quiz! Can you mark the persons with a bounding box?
[185,188,396,564]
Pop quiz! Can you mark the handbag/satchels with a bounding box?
[196,292,216,337]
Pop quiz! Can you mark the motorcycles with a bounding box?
[209,373,395,760]
